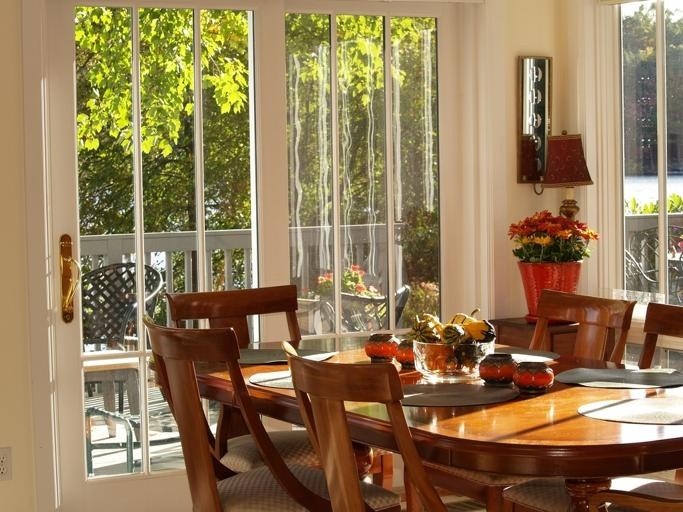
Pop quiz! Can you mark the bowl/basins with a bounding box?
[410,340,493,381]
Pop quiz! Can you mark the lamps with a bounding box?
[519,55,593,194]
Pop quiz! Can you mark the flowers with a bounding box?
[507,208,601,264]
